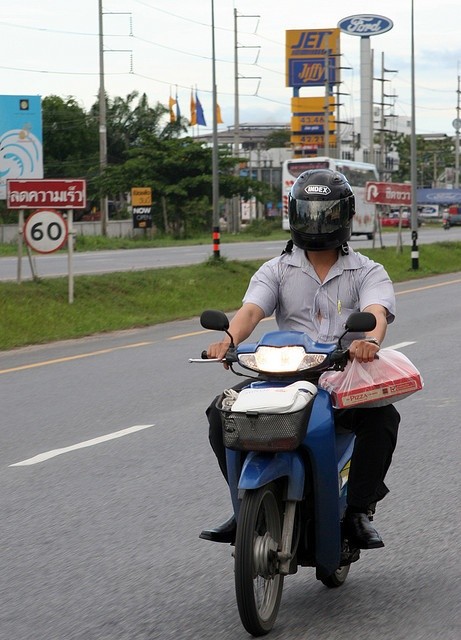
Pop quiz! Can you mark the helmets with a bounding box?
[281,169,355,257]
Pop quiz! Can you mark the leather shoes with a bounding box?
[343,512,386,550]
[200,514,237,544]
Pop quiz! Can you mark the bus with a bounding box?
[280,156,380,240]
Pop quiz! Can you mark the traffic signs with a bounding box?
[6,177,87,209]
[453,118,461,130]
[365,181,411,206]
[23,208,69,255]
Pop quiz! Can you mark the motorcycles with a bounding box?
[186,335,380,636]
[442,217,452,230]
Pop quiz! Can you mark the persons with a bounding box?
[198,170,400,550]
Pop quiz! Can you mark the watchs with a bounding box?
[361,336,381,347]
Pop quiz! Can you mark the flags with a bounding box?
[169,97,177,123]
[196,94,207,126]
[190,94,197,126]
[176,96,181,123]
[216,104,225,124]
